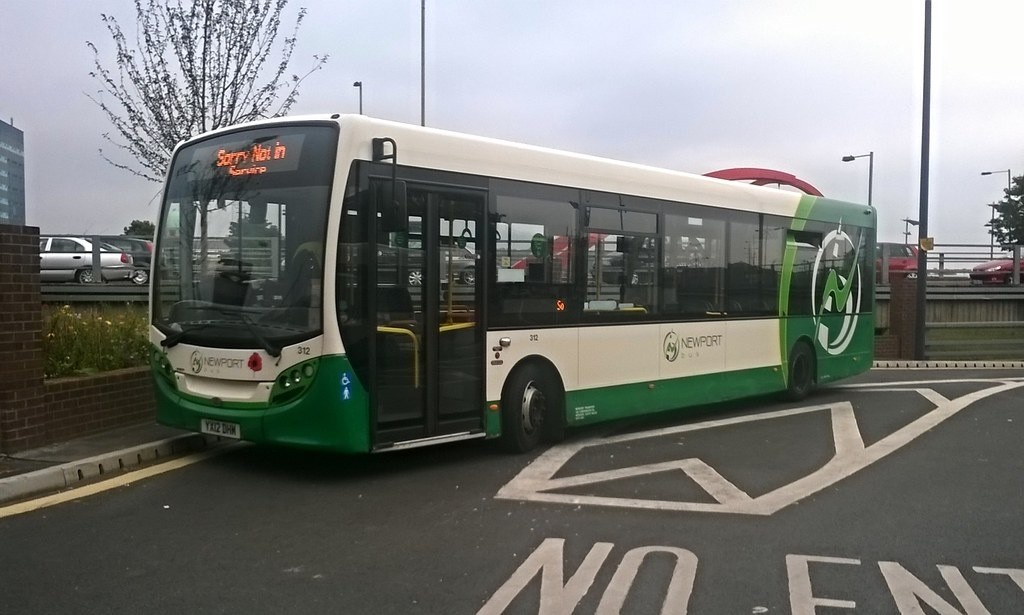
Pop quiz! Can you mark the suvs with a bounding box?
[398,241,477,286]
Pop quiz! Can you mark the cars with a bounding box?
[876,243,921,287]
[40,235,132,285]
[969,246,1024,285]
[89,235,156,285]
[595,243,701,284]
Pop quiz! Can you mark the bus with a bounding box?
[146,111,876,457]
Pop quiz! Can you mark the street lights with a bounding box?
[982,170,1012,253]
[354,82,363,116]
[840,151,873,204]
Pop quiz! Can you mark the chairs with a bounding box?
[584,301,770,313]
